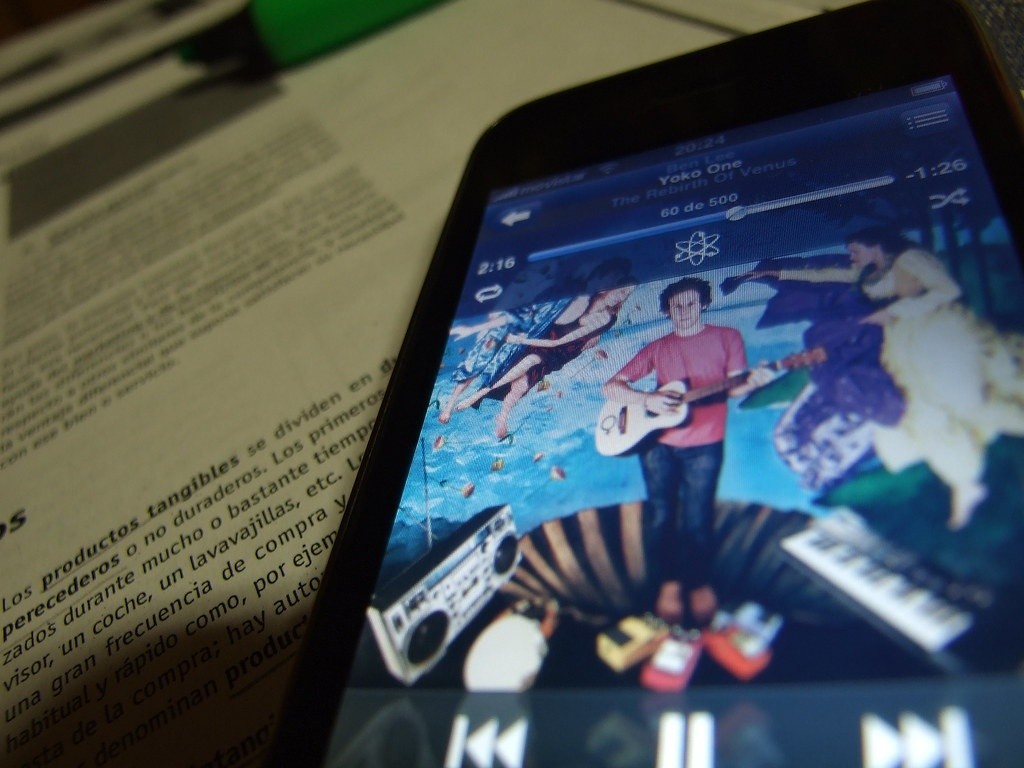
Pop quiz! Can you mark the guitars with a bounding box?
[594,344,829,458]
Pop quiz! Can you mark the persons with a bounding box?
[439,257,638,437]
[602,279,772,618]
[736,228,1024,531]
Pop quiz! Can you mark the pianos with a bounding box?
[771,524,982,670]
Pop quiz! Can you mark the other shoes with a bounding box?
[690,588,718,619]
[948,484,987,531]
[658,581,683,614]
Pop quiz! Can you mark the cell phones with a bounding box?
[262,0,1024,768]
